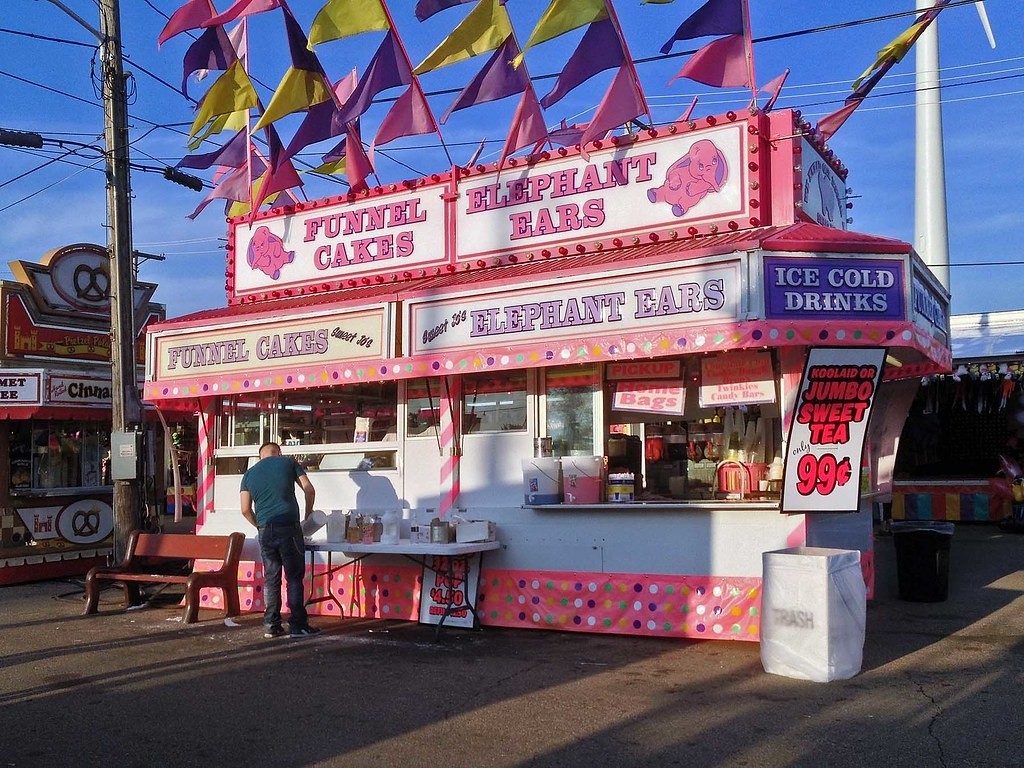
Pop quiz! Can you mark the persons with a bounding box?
[240,442,320,638]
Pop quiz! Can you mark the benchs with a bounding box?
[80,529,247,624]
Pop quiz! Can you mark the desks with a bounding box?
[304,539,501,644]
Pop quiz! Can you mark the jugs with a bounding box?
[301,510,327,536]
[327,510,345,543]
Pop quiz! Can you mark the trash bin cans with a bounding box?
[760,546,867,683]
[890,521,954,603]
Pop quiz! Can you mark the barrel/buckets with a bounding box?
[521,457,560,505]
[561,456,601,505]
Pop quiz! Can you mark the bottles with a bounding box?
[346,510,403,544]
[410,516,419,544]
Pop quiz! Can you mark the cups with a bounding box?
[723,407,766,463]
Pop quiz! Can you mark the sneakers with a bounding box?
[291,627,321,637]
[265,628,285,638]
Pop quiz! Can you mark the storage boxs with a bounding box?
[455,521,497,543]
[759,549,867,682]
